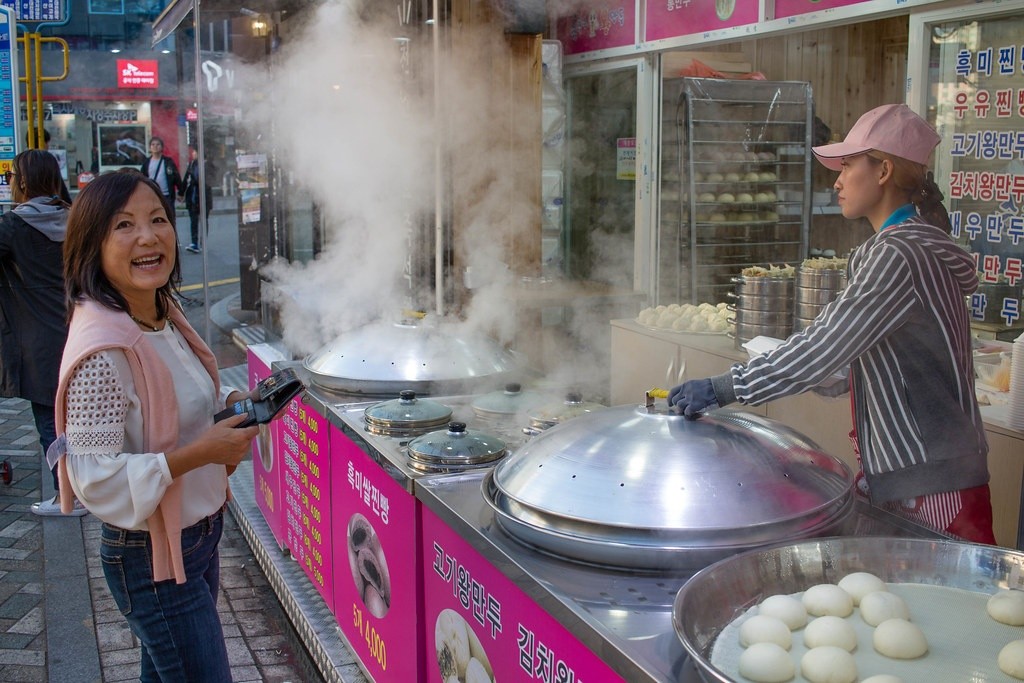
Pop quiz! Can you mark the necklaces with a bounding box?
[127,310,175,333]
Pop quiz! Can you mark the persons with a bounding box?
[140,136,183,232]
[177,144,217,253]
[667,102,998,547]
[0,148,90,517]
[46,166,260,683]
[25,126,52,150]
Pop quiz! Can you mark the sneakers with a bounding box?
[185,243,199,253]
[30,494,87,516]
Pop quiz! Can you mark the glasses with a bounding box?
[5,171,23,185]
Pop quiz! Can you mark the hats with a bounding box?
[811,104,942,172]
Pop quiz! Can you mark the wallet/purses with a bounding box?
[213,367,304,430]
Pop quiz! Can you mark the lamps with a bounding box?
[251,16,267,37]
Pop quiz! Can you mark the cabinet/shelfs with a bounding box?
[659,79,812,307]
[609,318,1024,549]
[777,147,842,215]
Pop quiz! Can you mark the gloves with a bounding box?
[667,377,718,417]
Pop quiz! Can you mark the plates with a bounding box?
[786,190,833,206]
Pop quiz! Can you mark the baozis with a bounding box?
[435,609,495,683]
[346,513,391,618]
[638,302,736,333]
